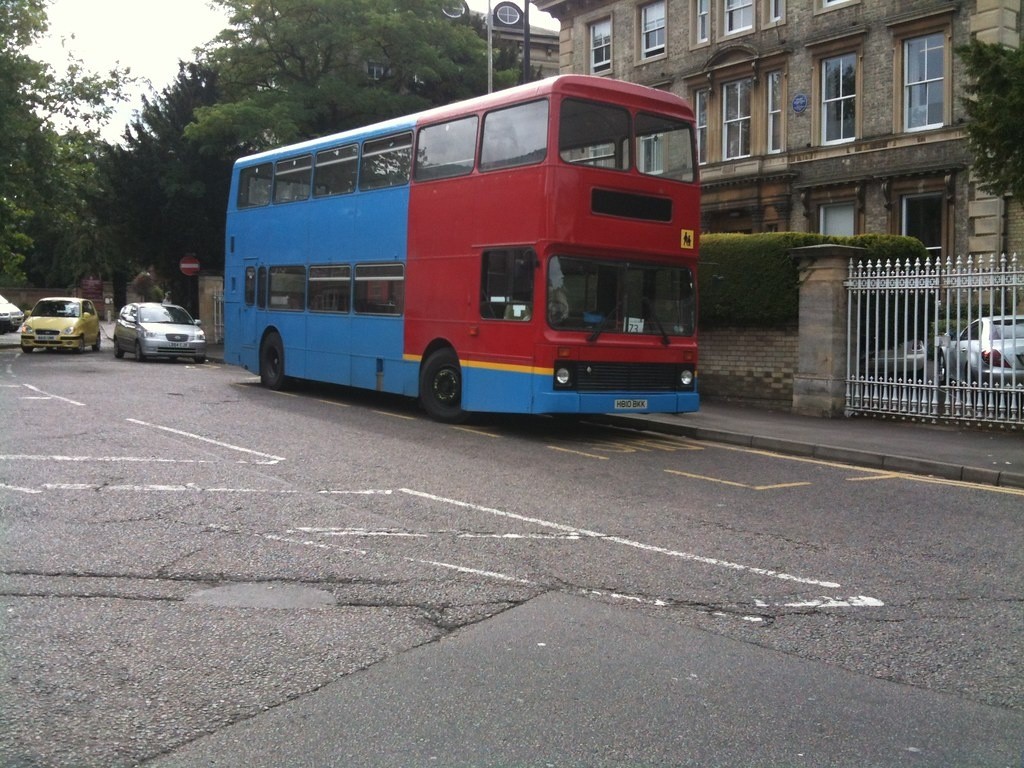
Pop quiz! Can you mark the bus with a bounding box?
[222,74,706,423]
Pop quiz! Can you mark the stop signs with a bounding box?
[181,256,200,276]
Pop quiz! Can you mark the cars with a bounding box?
[112,302,207,365]
[19,296,101,353]
[939,315,1024,392]
[860,335,926,376]
[1,295,24,330]
